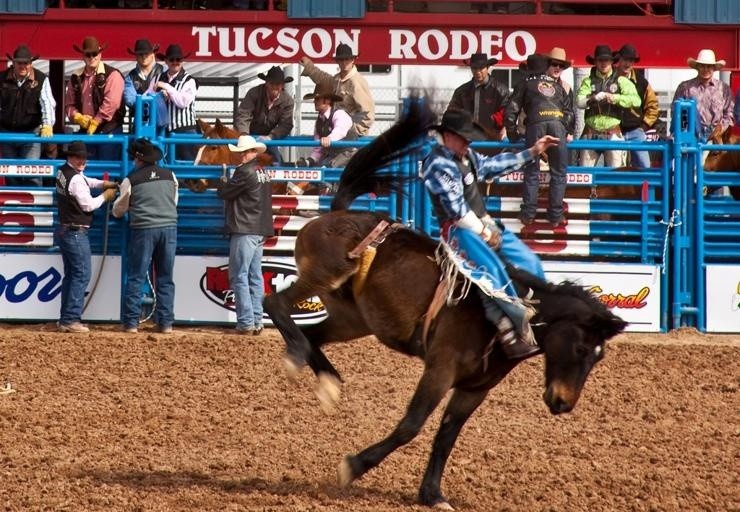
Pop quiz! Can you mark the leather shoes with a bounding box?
[125,327,139,332]
[159,326,172,334]
[518,214,534,226]
[225,328,253,335]
[551,216,561,228]
[253,329,261,335]
[503,343,541,360]
[58,323,90,333]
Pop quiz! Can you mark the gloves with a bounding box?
[593,91,607,103]
[72,112,92,129]
[40,125,54,137]
[86,118,100,135]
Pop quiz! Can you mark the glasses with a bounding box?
[551,62,564,67]
[85,52,97,58]
[168,58,181,62]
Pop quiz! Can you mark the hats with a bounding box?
[428,108,490,142]
[6,46,40,63]
[549,47,574,66]
[586,45,621,64]
[127,40,160,55]
[332,41,361,60]
[126,138,163,163]
[463,54,498,68]
[687,49,726,70]
[257,66,294,83]
[303,83,343,101]
[227,135,267,154]
[63,142,95,156]
[156,44,190,60]
[613,45,641,63]
[72,36,108,53]
[519,54,552,75]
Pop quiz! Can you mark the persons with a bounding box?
[110,134,182,335]
[421,104,562,351]
[50,136,120,336]
[213,128,277,336]
[3,38,740,258]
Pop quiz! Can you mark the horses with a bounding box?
[189,116,310,196]
[259,78,629,512]
[439,96,740,264]
